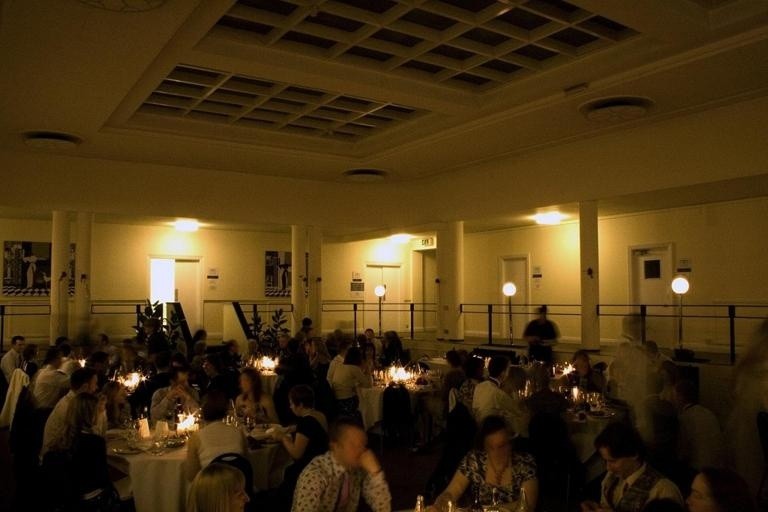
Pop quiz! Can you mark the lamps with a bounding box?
[671,277,690,295]
[578,98,652,121]
[24,133,84,156]
[375,285,386,296]
[342,169,388,183]
[502,283,516,297]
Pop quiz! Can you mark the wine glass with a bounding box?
[555,360,574,371]
[517,386,601,409]
[124,408,272,456]
[373,367,428,387]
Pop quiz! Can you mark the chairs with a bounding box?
[381,385,410,433]
[203,452,255,509]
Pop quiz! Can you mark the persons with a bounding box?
[1,303,767,512]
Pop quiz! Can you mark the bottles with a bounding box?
[414,486,529,512]
[521,353,556,377]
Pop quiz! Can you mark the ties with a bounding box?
[19,354,21,368]
[335,472,349,512]
